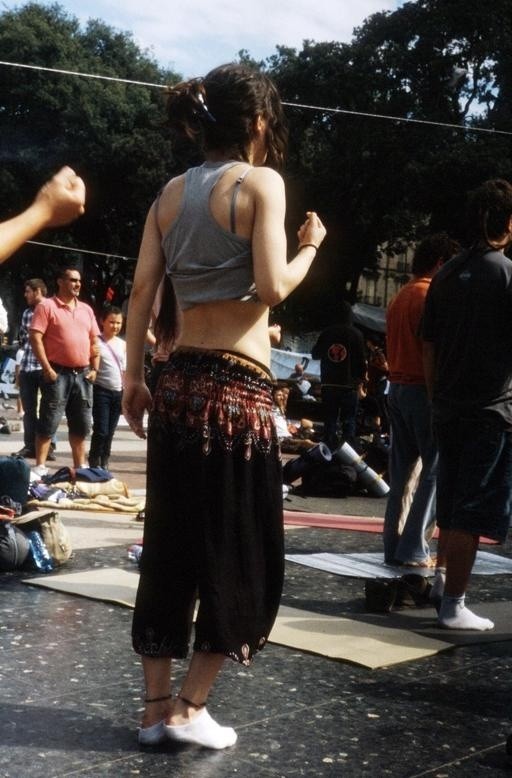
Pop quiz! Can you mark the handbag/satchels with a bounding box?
[0,514,73,566]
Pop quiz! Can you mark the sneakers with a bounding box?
[12,441,110,483]
[365,574,436,614]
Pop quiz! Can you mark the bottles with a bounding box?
[30,531,53,572]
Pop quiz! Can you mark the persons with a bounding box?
[382,178,512,632]
[271,298,390,497]
[0,262,127,482]
[0,164,90,264]
[120,61,328,751]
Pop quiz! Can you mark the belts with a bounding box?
[50,360,93,374]
